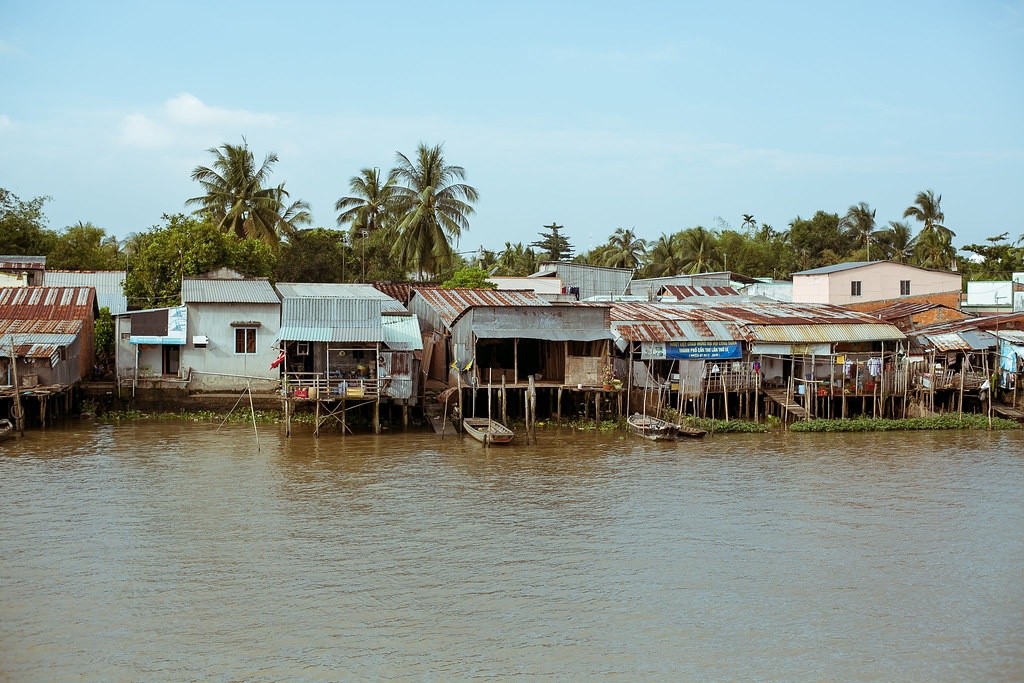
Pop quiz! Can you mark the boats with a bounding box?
[628,414,679,441]
[672,423,707,438]
[462,417,515,444]
[0,419,13,441]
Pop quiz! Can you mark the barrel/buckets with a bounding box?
[799,384,805,394]
[308,387,317,399]
[357,364,368,377]
